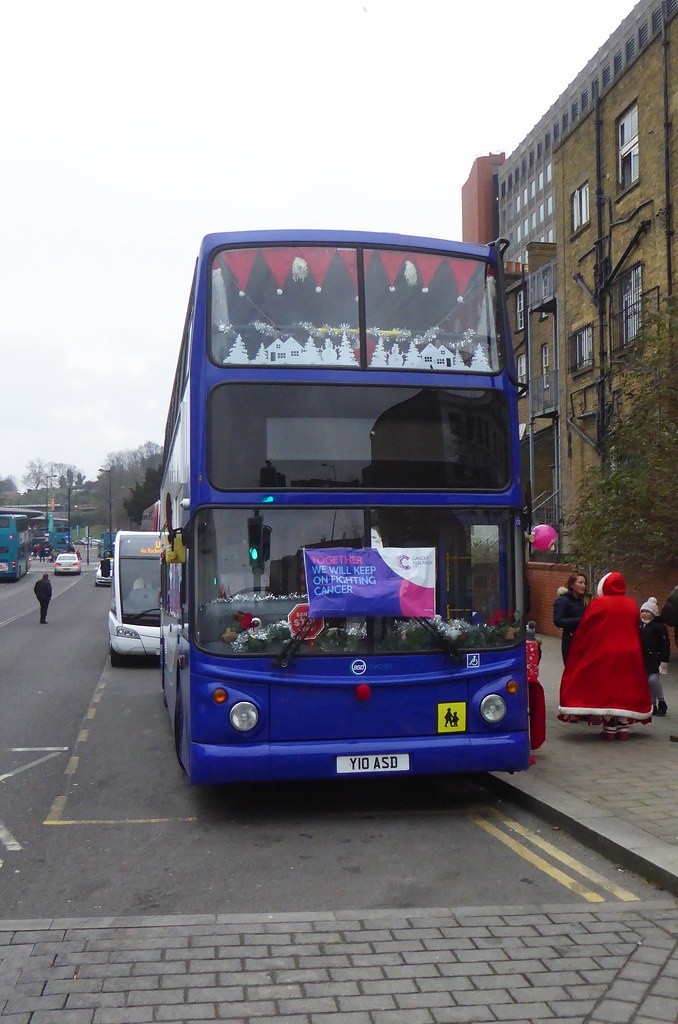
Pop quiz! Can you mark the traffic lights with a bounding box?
[248,518,262,565]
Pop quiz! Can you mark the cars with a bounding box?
[94,560,113,587]
[54,554,82,576]
[89,539,102,546]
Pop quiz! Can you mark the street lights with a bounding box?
[46,475,57,532]
[321,464,337,482]
[99,469,111,550]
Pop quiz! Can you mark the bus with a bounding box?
[159,228,533,789]
[0,515,29,582]
[31,518,73,557]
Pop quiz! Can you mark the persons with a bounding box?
[558,571,654,741]
[34,574,52,624]
[526,621,542,679]
[553,572,594,668]
[65,544,75,553]
[638,597,671,716]
[31,546,58,563]
[76,551,80,560]
[661,585,678,742]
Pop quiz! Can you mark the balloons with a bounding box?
[531,525,557,550]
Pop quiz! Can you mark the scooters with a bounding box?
[101,531,161,669]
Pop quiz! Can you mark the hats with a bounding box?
[536,637,543,659]
[526,621,536,639]
[640,597,661,617]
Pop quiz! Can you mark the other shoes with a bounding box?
[40,620,48,624]
[657,701,667,716]
[652,706,657,715]
[617,726,629,741]
[670,734,677,742]
[600,726,618,741]
